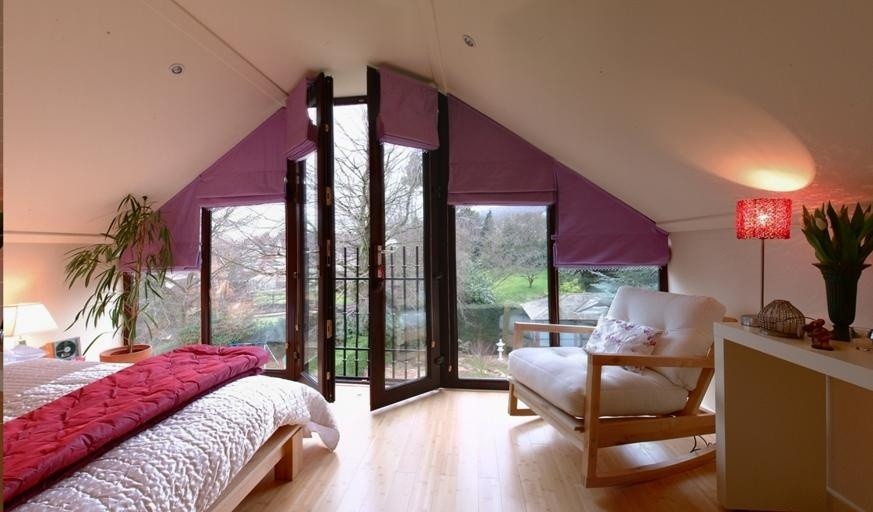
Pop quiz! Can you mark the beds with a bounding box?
[1,344,342,512]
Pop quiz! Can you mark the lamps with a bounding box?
[735,195,792,327]
[3,301,60,362]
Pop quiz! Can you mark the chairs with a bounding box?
[503,283,738,491]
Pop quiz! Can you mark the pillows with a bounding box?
[581,311,666,376]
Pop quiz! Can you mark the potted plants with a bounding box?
[61,191,178,365]
[799,200,872,342]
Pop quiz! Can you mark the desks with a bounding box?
[710,320,873,512]
